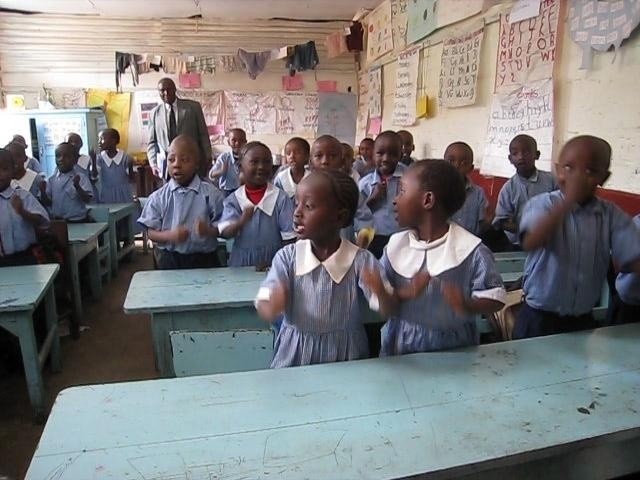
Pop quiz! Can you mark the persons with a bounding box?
[378,160,507,359]
[512,136,640,340]
[354,130,412,260]
[10,134,41,174]
[209,128,248,200]
[393,130,415,176]
[89,128,133,251]
[38,142,94,304]
[5,143,41,206]
[491,134,560,251]
[136,134,225,269]
[443,141,488,247]
[53,132,93,176]
[0,149,51,369]
[254,170,398,368]
[309,135,374,245]
[218,141,297,267]
[606,213,640,326]
[339,143,361,184]
[147,78,212,187]
[274,137,311,206]
[352,138,376,178]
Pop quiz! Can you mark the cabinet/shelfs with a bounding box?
[1,108,107,182]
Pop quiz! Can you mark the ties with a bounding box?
[168,104,177,144]
